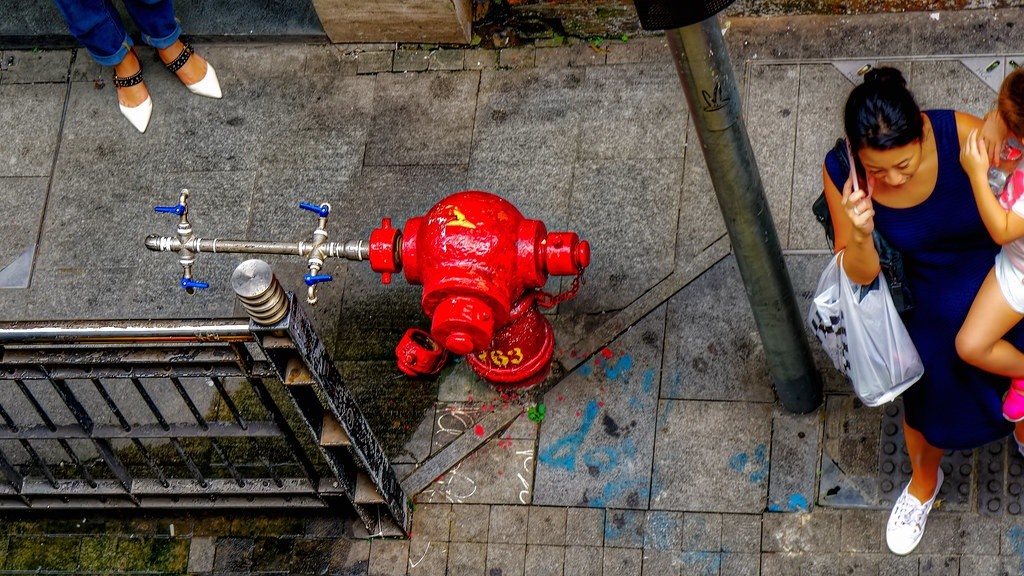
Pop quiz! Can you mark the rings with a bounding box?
[854,207,860,215]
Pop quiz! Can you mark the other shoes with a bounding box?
[1001,378,1024,422]
[158,40,222,99]
[113,50,153,134]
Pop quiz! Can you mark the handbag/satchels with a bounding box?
[807,248,924,407]
[812,138,917,312]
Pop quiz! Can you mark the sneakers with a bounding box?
[886,466,944,556]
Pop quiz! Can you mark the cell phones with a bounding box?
[844,137,868,201]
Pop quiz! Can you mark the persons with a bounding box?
[55,0,223,133]
[822,67,1024,557]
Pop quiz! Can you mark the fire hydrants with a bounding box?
[142,185,592,388]
